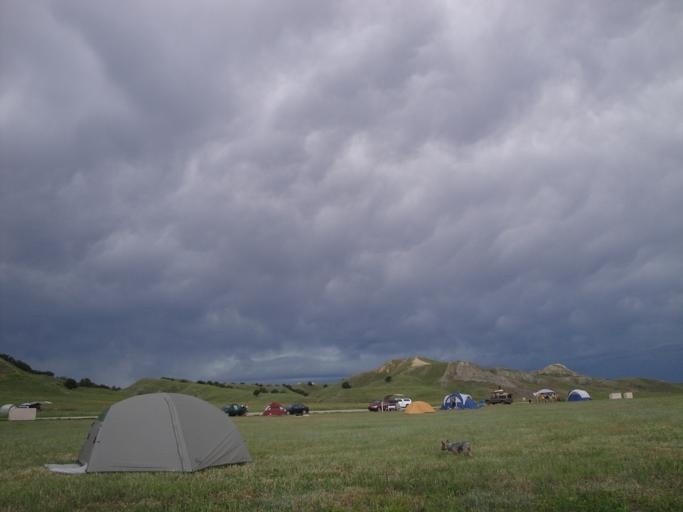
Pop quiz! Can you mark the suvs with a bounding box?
[484,391,513,405]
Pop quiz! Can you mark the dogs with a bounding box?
[440,439,473,457]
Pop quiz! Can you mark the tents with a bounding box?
[405,401,436,414]
[567,389,592,401]
[78,393,254,474]
[442,391,479,410]
[262,402,290,417]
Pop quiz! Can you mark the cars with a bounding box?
[283,402,309,414]
[367,398,412,412]
[222,402,247,416]
